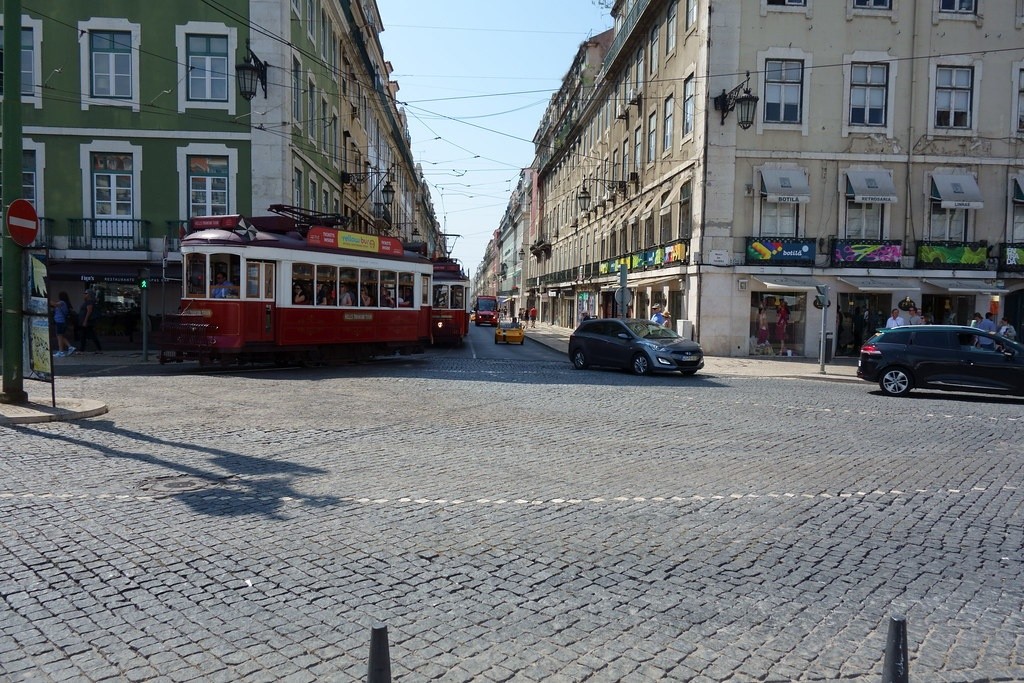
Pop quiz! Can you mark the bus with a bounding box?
[148,204,472,370]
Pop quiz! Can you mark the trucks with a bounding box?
[474,295,500,326]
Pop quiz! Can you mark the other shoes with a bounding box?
[75,351,84,355]
[94,351,104,354]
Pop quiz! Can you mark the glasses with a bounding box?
[361,290,365,291]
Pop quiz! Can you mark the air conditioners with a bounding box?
[568,217,576,226]
[391,172,396,181]
[352,107,358,117]
[628,89,637,103]
[616,105,625,118]
[605,192,612,201]
[552,228,557,236]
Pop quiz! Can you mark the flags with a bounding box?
[233,217,258,241]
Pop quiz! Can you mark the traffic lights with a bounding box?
[141,279,148,288]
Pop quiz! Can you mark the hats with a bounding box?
[651,303,660,309]
[660,311,669,317]
[581,311,588,314]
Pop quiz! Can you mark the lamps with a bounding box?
[236,41,268,102]
[392,220,422,241]
[575,178,626,212]
[342,168,395,205]
[518,243,533,260]
[715,71,759,130]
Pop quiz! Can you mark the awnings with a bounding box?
[924,277,1010,292]
[840,275,921,291]
[753,275,831,289]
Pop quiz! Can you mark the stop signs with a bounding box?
[6,198,38,248]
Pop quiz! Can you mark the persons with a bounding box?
[94,286,103,304]
[340,283,357,306]
[970,312,1016,349]
[380,286,395,308]
[530,306,537,328]
[755,301,789,352]
[317,283,332,304]
[211,272,235,298]
[503,306,507,319]
[399,289,413,306]
[76,289,103,354]
[886,309,904,328]
[523,310,529,329]
[908,307,933,325]
[361,287,370,306]
[651,305,664,325]
[50,291,76,357]
[293,281,309,305]
[661,312,671,330]
[581,312,590,321]
[518,308,524,321]
[511,318,520,328]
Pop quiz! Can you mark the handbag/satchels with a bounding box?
[86,302,101,321]
[65,310,80,326]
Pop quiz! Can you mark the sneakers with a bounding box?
[52,352,65,358]
[67,347,77,357]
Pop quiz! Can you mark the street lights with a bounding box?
[815,285,825,304]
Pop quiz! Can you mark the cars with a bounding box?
[856,324,1024,396]
[495,323,525,345]
[471,310,475,321]
[568,318,705,376]
